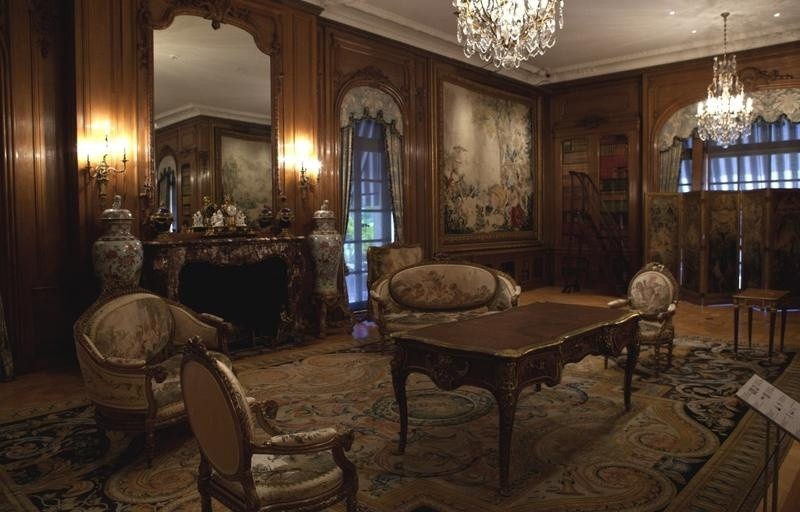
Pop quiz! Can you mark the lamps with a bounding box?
[451,0,564,71]
[293,155,319,203]
[85,120,129,211]
[696,13,754,149]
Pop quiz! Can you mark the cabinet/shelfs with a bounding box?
[552,116,640,288]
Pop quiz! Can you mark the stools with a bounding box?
[732,289,790,362]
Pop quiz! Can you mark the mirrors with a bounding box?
[143,0,283,234]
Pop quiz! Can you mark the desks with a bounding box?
[390,302,644,497]
[144,236,308,344]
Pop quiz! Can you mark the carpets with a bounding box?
[0,337,800,512]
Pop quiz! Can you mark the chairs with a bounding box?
[370,262,520,355]
[367,240,423,325]
[181,336,359,512]
[604,262,679,378]
[73,288,234,469]
